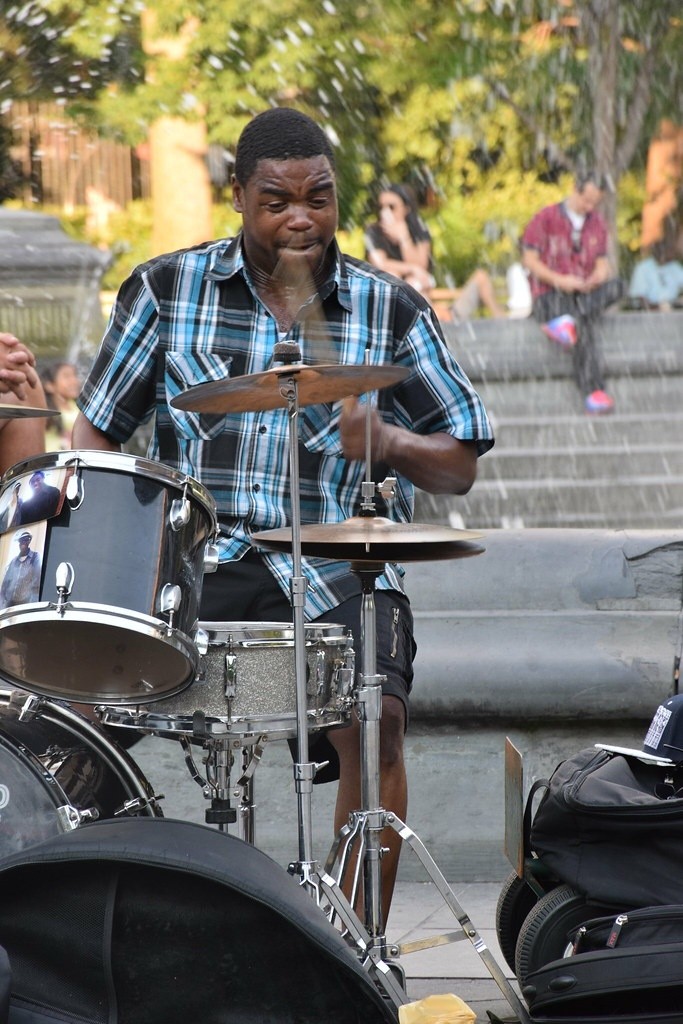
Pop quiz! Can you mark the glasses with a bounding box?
[380,203,397,211]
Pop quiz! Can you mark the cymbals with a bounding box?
[249,515,487,562]
[170,365,412,414]
[0,403,62,420]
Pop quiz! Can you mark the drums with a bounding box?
[0,689,166,863]
[98,621,357,740]
[0,447,221,707]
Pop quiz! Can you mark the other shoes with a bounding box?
[584,391,614,414]
[540,314,577,348]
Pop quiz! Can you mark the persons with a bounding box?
[0,333,89,477]
[69,109,495,931]
[362,180,437,298]
[519,170,624,414]
